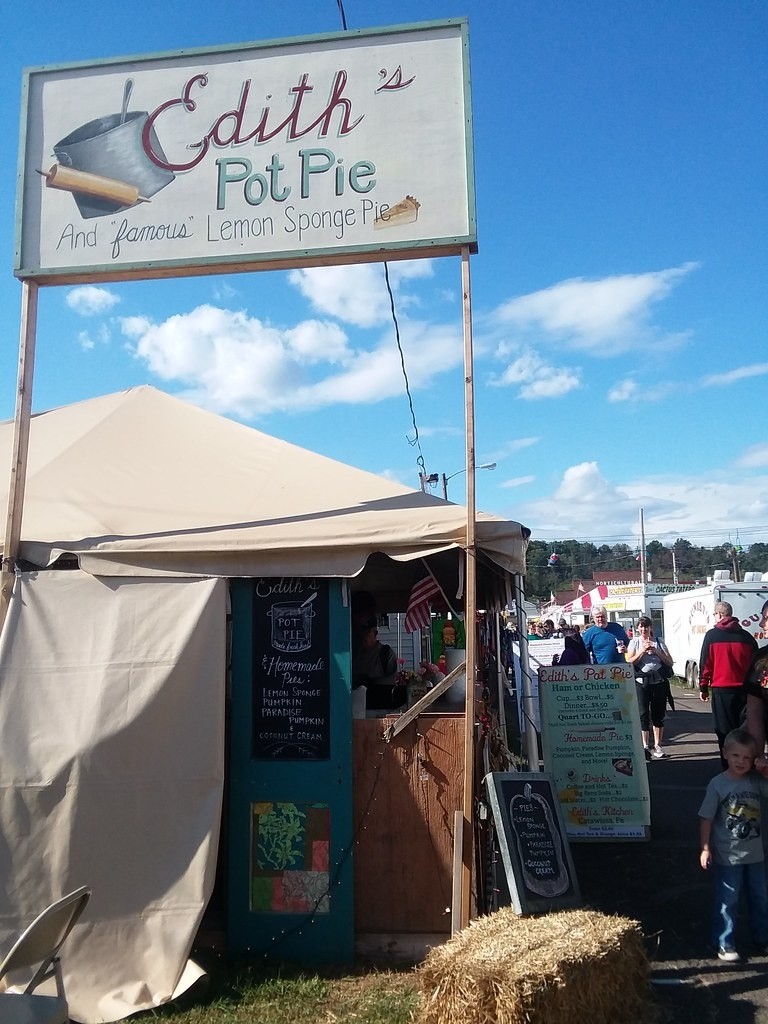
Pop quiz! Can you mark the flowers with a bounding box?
[391,657,446,696]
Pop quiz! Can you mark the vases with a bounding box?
[405,685,428,712]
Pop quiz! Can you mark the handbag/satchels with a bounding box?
[656,637,674,678]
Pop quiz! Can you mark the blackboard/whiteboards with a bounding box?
[483,771,585,917]
[251,577,333,763]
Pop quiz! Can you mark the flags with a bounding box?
[404,557,443,634]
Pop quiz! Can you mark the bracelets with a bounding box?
[701,848,710,851]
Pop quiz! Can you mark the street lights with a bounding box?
[438,461,498,505]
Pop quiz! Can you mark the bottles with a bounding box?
[443,621,455,655]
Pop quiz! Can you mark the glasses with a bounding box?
[713,612,723,616]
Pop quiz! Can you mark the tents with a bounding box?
[0,380,540,981]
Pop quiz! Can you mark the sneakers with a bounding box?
[653,746,666,757]
[644,745,652,761]
[709,945,740,961]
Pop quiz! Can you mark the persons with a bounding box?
[695,728,768,961]
[697,601,759,771]
[551,628,593,667]
[502,618,582,643]
[353,614,398,709]
[581,606,630,664]
[627,616,674,760]
[743,643,768,776]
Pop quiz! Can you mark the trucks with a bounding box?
[663,580,768,692]
